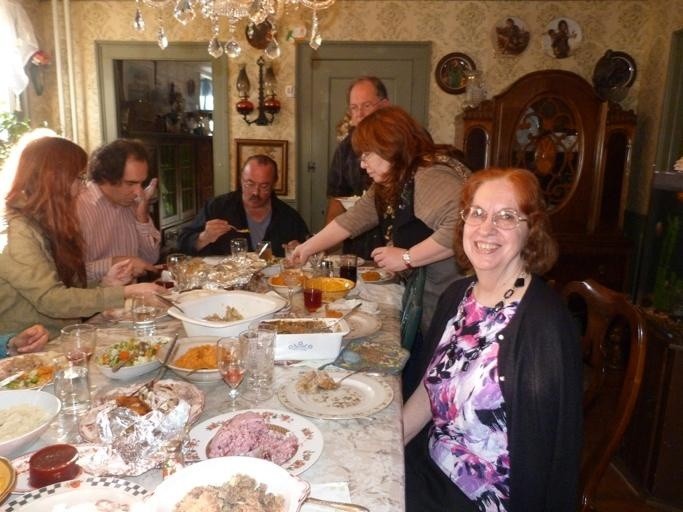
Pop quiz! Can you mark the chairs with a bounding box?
[558,279,648,511]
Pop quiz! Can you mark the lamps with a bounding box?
[236,57,281,125]
[133,0,336,60]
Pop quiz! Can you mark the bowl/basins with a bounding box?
[257,315,350,360]
[334,196,361,210]
[166,290,289,338]
[298,277,356,302]
[268,271,315,294]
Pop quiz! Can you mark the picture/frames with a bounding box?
[235,139,288,195]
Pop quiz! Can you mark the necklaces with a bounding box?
[446,270,525,370]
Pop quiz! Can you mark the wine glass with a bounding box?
[132,293,157,338]
[279,256,301,313]
[57,324,98,441]
[167,254,192,293]
[218,331,276,413]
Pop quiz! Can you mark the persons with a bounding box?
[280,104,480,404]
[176,154,311,257]
[76,138,163,277]
[400,168,587,511]
[0,324,50,358]
[0,135,172,339]
[324,75,393,261]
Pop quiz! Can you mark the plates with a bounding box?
[156,335,239,383]
[184,408,326,476]
[1,457,16,502]
[1,351,60,390]
[152,455,301,512]
[96,337,175,382]
[274,363,395,421]
[342,310,384,340]
[1,472,153,511]
[1,388,62,454]
[245,20,278,50]
[358,266,396,284]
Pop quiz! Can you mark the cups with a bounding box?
[231,239,272,265]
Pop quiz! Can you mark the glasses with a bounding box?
[459,205,527,230]
[76,174,88,186]
[349,98,383,112]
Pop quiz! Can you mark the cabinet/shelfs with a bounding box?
[610,305,682,506]
[157,138,197,229]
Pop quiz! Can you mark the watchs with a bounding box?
[401,250,412,269]
